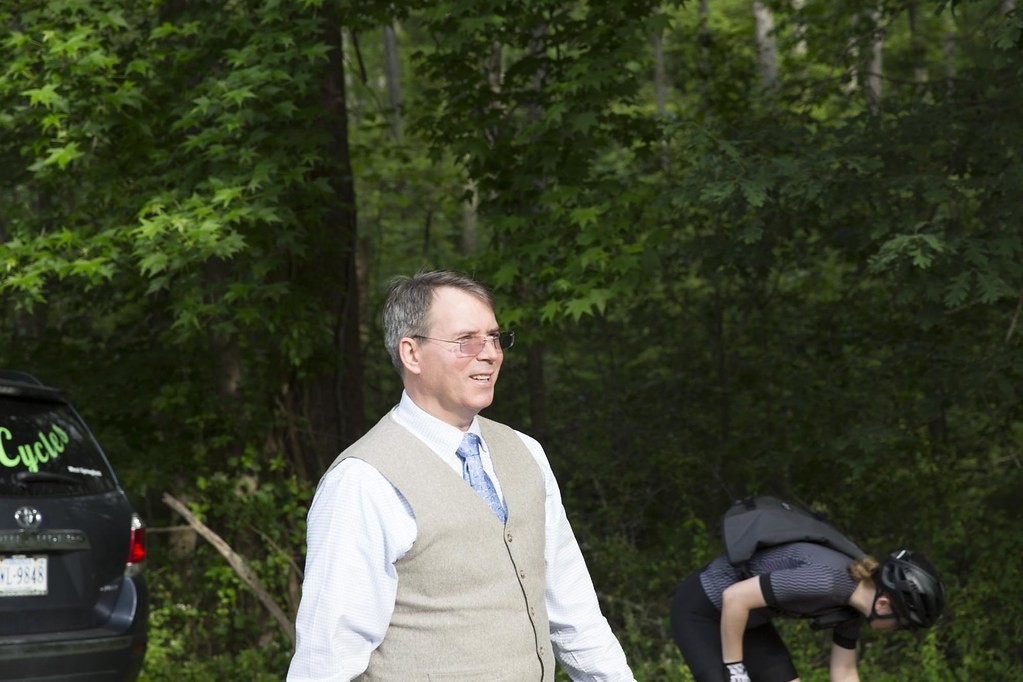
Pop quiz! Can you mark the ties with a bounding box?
[456,433,507,525]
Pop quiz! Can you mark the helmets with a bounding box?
[880,549,945,629]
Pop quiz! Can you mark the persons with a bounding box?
[284,266,642,682]
[668,539,945,682]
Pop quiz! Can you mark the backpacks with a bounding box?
[722,496,866,629]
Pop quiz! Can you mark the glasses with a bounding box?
[890,599,911,632]
[412,331,515,355]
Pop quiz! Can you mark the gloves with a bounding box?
[725,662,750,682]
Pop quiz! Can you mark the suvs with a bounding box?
[0,368,151,682]
